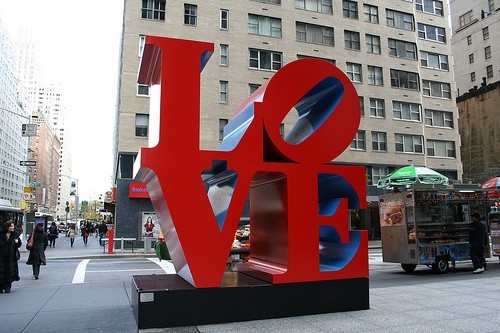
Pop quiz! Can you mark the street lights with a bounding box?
[57,174,78,235]
[65,201,69,237]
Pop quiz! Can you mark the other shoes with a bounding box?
[482,267,484,271]
[35,277,39,279]
[473,268,482,274]
[0,290,3,293]
[5,290,10,293]
[50,245,51,247]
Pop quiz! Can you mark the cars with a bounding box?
[46,218,85,233]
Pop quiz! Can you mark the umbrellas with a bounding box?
[377,165,450,189]
[482,177,500,189]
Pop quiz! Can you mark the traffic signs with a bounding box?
[20,160,37,166]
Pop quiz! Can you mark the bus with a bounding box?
[0,198,54,237]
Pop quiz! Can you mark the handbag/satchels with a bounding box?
[16,250,20,260]
[84,232,88,236]
[26,230,34,250]
[75,228,77,235]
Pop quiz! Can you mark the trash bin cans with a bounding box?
[144,237,151,254]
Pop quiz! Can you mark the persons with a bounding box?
[80,221,98,245]
[470,212,486,272]
[67,223,78,247]
[25,223,48,279]
[0,220,23,294]
[49,222,58,248]
[143,217,155,237]
[98,221,108,246]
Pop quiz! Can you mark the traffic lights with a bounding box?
[65,207,69,212]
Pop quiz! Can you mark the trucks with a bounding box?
[378,179,500,274]
